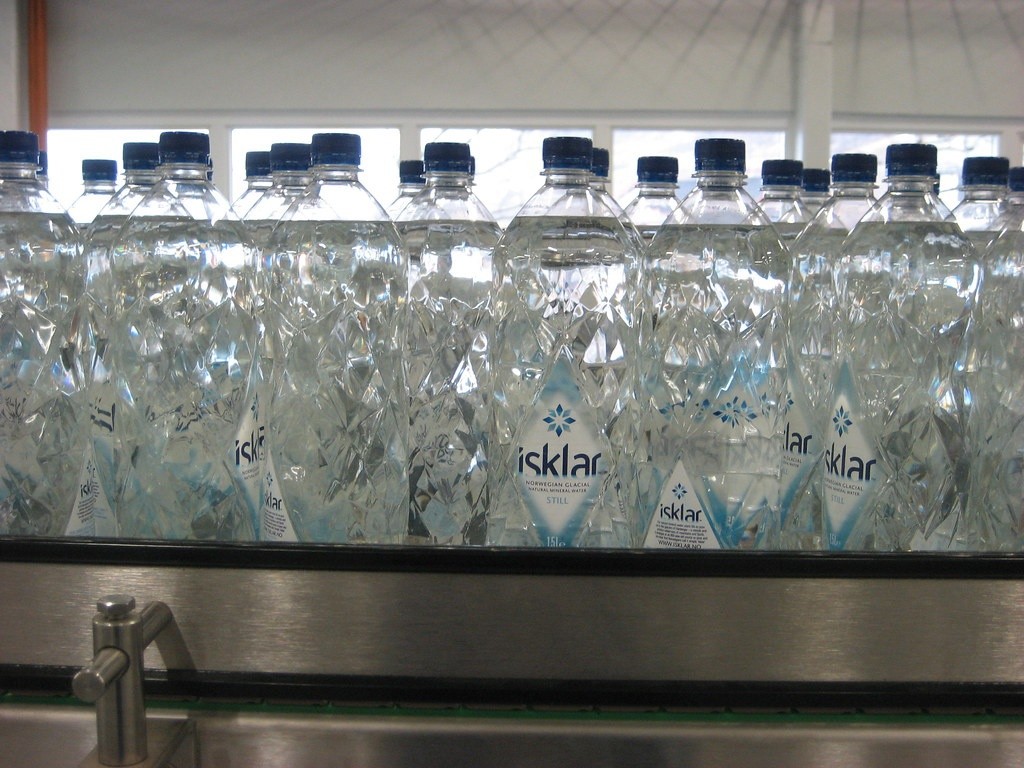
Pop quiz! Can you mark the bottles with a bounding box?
[0,128,1023,556]
